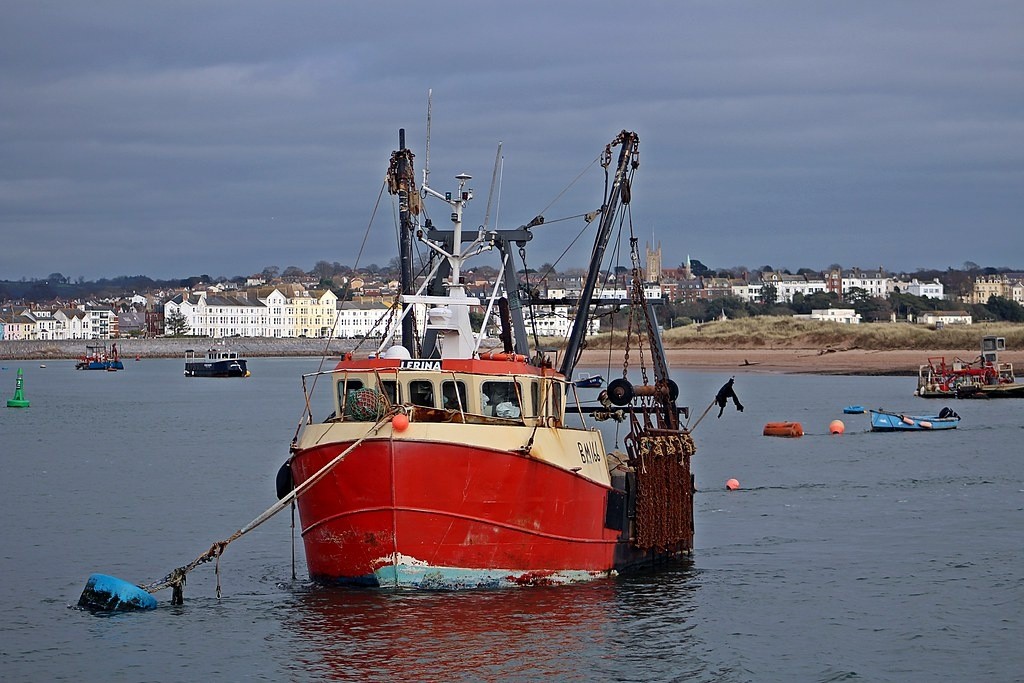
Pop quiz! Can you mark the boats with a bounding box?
[914,327,1024,398]
[869,406,963,432]
[75,321,125,372]
[274,85,740,591]
[184,348,251,378]
[843,404,866,414]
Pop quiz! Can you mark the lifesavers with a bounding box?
[956,383,962,390]
[973,382,980,389]
[655,379,679,403]
[607,379,633,406]
